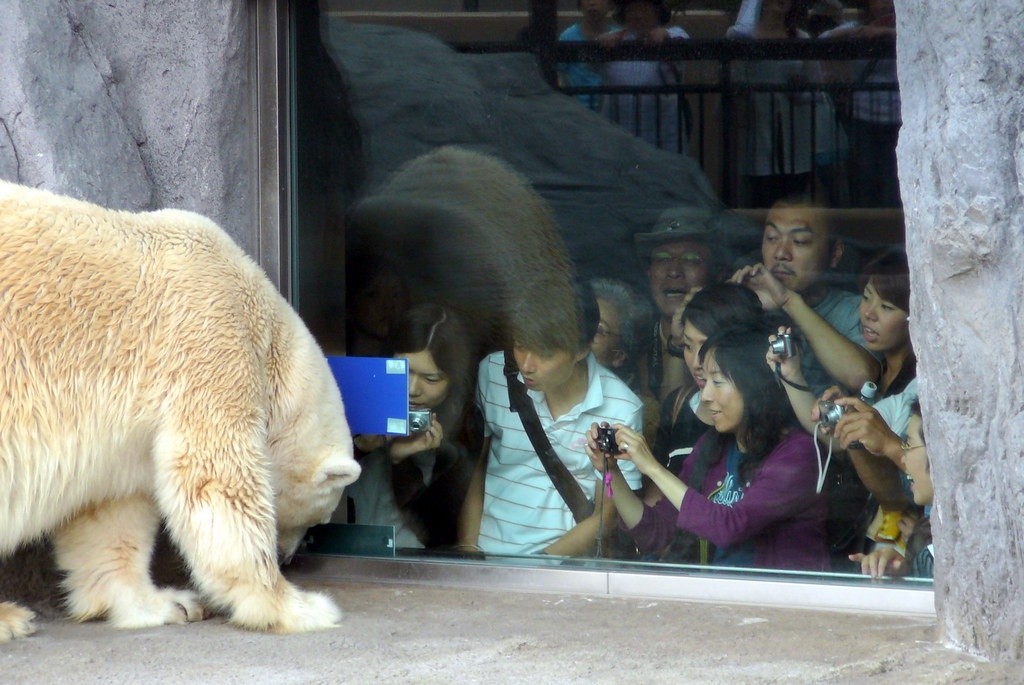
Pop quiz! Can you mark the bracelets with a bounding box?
[667,335,685,359]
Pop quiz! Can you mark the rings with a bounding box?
[622,443,629,452]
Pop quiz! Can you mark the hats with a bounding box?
[633,205,730,278]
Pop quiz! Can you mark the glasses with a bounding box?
[593,320,623,345]
[900,441,924,457]
[648,253,711,273]
[623,4,657,16]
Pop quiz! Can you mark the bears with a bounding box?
[0,177,361,642]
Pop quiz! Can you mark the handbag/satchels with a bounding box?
[574,475,645,561]
[821,452,898,556]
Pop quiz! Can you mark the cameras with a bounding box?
[408,408,433,434]
[771,334,796,360]
[818,400,845,430]
[597,427,622,455]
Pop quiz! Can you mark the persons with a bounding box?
[290,0,932,577]
